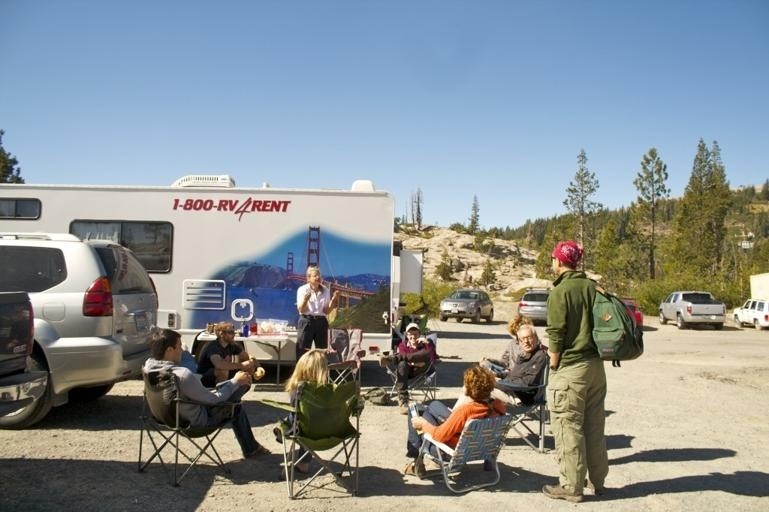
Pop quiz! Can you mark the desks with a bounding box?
[197,332,289,387]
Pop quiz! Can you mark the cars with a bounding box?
[619,297,643,326]
[0,292,49,420]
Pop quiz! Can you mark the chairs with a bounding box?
[280,360,361,498]
[138,365,241,488]
[327,329,362,388]
[386,333,438,406]
[414,415,514,497]
[483,355,551,452]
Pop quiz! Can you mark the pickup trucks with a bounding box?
[659,290,726,329]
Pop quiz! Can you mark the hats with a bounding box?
[406,322,420,332]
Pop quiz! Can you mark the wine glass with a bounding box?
[383,351,390,359]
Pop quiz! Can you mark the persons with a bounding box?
[139,327,273,459]
[403,363,506,477]
[295,263,341,362]
[198,322,258,390]
[378,321,436,415]
[540,239,609,503]
[273,348,331,474]
[479,312,543,379]
[451,324,547,416]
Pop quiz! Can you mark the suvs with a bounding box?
[733,298,769,330]
[518,287,549,324]
[0,232,158,429]
[439,289,494,322]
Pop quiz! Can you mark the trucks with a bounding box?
[0,174,402,378]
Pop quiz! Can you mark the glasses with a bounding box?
[550,256,556,262]
[222,329,235,334]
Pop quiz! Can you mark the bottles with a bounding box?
[243,324,249,337]
[250,322,257,336]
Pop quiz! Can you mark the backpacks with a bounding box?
[590,286,643,368]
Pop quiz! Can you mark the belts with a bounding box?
[302,316,324,319]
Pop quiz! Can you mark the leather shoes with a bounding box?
[585,480,604,495]
[542,485,583,502]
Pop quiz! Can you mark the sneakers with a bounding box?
[398,400,409,414]
[380,356,391,367]
[402,463,427,476]
[296,463,309,473]
[251,447,270,458]
[273,427,283,443]
